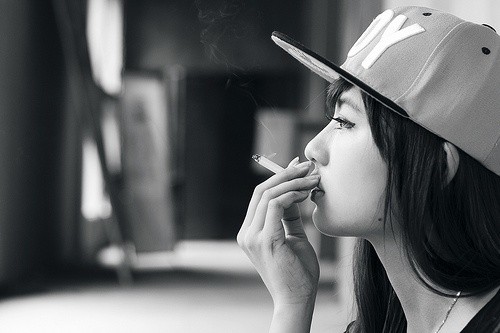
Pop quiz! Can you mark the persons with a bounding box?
[236,6,500,333]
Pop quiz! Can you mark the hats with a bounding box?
[272,6,499,176]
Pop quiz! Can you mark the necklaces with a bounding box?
[435,291,461,333]
